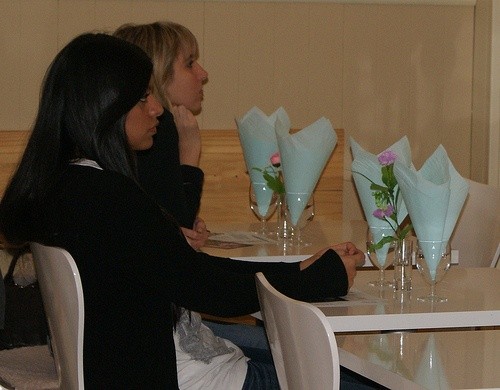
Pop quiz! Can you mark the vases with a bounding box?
[275,200,300,243]
[396,238,416,292]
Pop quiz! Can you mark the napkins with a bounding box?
[348,132,411,267]
[233,103,292,218]
[392,143,470,283]
[273,113,340,228]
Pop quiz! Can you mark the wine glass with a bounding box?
[283,192,315,247]
[415,239,452,304]
[366,225,399,289]
[249,182,279,236]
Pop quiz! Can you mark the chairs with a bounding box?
[450,177,500,267]
[28,242,86,390]
[257,271,341,390]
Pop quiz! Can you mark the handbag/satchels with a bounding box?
[0,249,50,350]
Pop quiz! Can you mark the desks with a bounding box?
[336,329,500,390]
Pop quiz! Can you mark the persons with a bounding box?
[0,20,392,390]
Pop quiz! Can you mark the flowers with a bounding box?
[344,150,414,255]
[249,152,286,205]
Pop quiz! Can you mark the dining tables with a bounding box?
[312,265,500,334]
[202,224,372,268]
